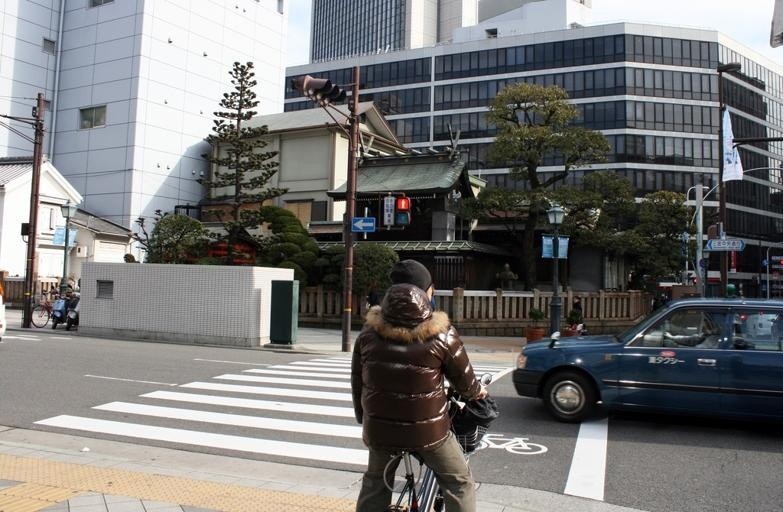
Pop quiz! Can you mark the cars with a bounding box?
[633,267,695,303]
[744,310,778,340]
[511,295,782,423]
[0,281,7,343]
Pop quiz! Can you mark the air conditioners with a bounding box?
[75,245,88,258]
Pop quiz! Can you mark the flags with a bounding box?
[721,109,745,182]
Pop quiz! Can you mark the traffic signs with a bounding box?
[707,240,745,253]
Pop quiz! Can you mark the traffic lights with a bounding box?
[780,260,783,265]
[296,75,346,109]
[395,195,410,227]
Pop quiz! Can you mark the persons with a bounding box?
[349,258,485,512]
[567,295,586,326]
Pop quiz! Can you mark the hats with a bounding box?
[390,259,432,292]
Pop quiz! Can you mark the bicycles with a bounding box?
[30,290,52,328]
[383,373,492,512]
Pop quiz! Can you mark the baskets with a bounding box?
[456,424,488,454]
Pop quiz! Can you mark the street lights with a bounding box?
[766,242,783,300]
[59,199,77,292]
[716,61,742,298]
[544,202,566,337]
[684,186,710,285]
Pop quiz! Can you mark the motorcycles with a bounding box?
[51,293,69,329]
[66,290,79,330]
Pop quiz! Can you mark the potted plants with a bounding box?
[563,308,588,338]
[525,307,546,344]
